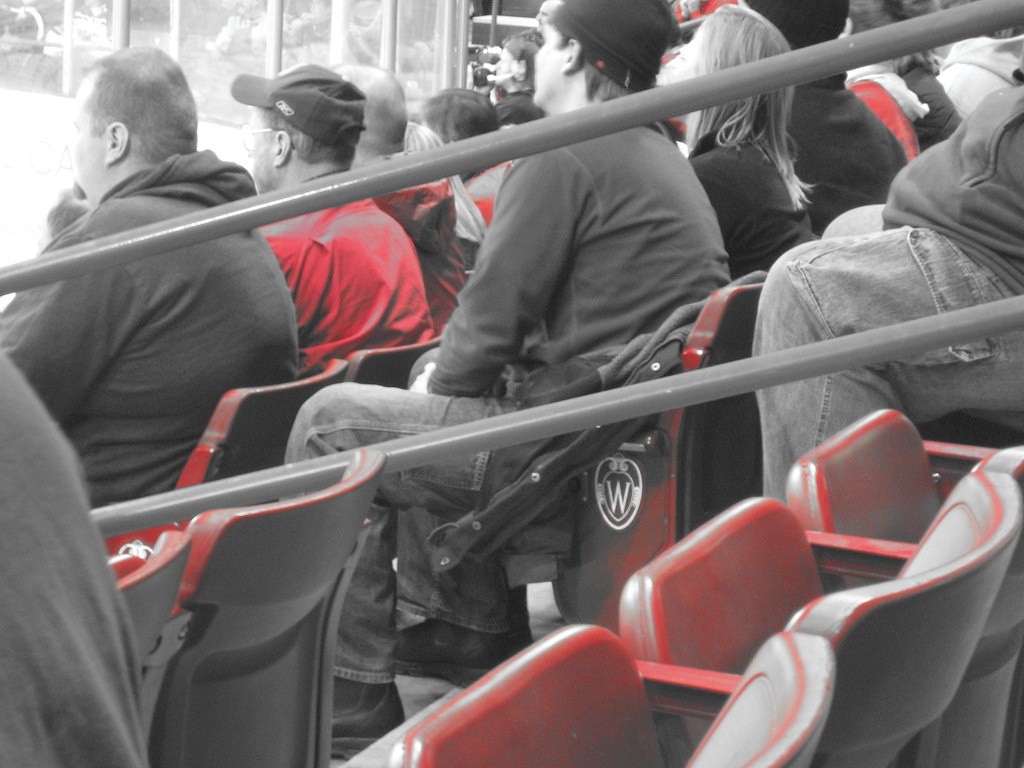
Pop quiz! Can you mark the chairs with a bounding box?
[100,273,1023,768]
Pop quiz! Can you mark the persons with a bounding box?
[751,83,1024,500]
[229,0,1024,365]
[0,42,305,511]
[278,0,742,762]
[0,348,154,768]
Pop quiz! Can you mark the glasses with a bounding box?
[241,122,272,150]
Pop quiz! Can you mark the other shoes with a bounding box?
[395,587,535,687]
[330,681,403,759]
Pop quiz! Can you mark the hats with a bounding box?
[745,0,850,49]
[541,0,681,91]
[504,40,540,74]
[230,64,366,145]
